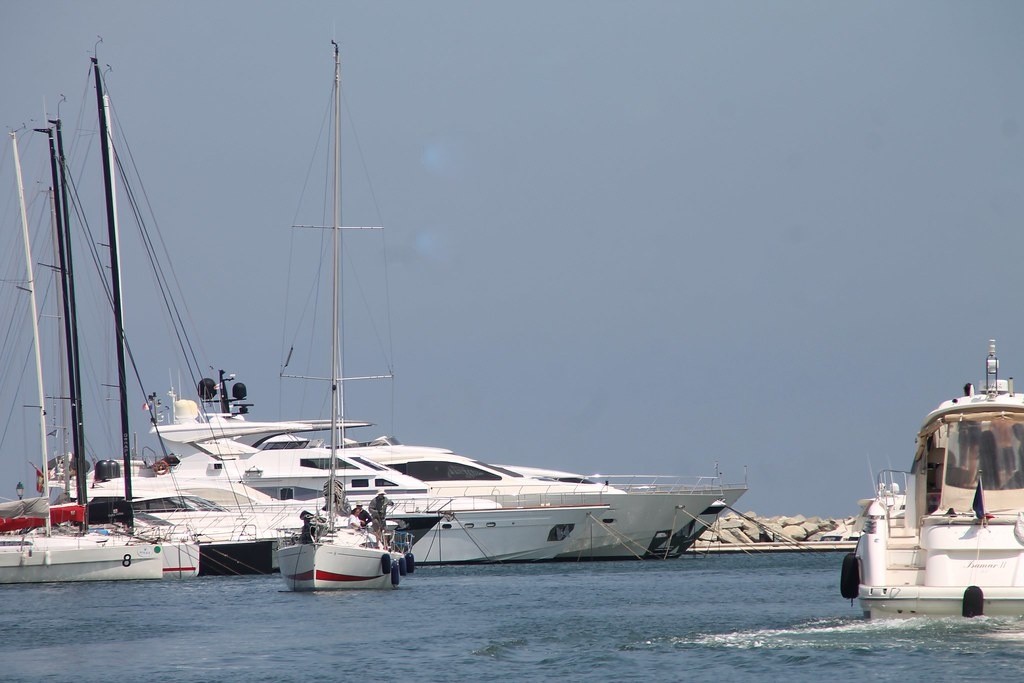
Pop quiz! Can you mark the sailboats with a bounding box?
[0,37,278,581]
[272,34,418,594]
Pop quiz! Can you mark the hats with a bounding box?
[356,501,363,505]
[377,488,387,494]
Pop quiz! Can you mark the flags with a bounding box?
[972,476,994,519]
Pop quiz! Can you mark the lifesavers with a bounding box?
[153,460,169,475]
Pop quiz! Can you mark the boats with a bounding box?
[839,338,1024,620]
[28,362,750,559]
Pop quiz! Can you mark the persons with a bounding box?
[351,500,371,525]
[350,508,365,531]
[368,488,394,532]
